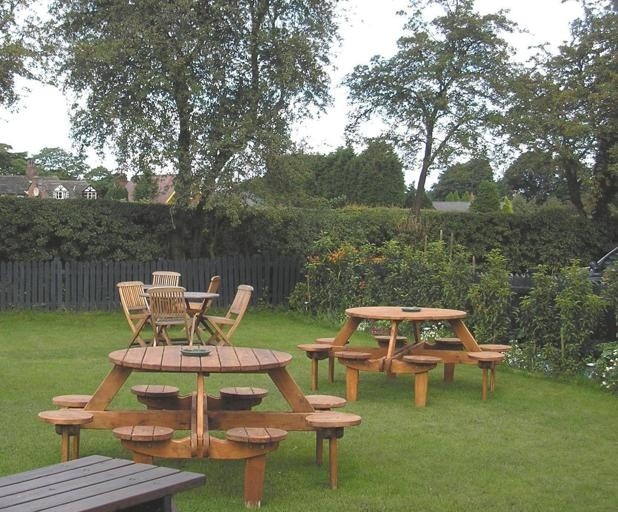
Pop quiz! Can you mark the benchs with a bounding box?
[334,347,437,409]
[303,388,361,492]
[112,419,289,507]
[467,338,513,404]
[37,384,94,464]
[298,333,350,388]
[131,386,268,411]
[374,326,467,383]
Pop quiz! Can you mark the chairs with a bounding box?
[115,281,175,349]
[182,275,222,343]
[150,286,205,350]
[195,280,255,347]
[150,271,182,289]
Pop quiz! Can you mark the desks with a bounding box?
[84,346,312,441]
[138,290,220,346]
[0,450,213,511]
[328,306,479,377]
